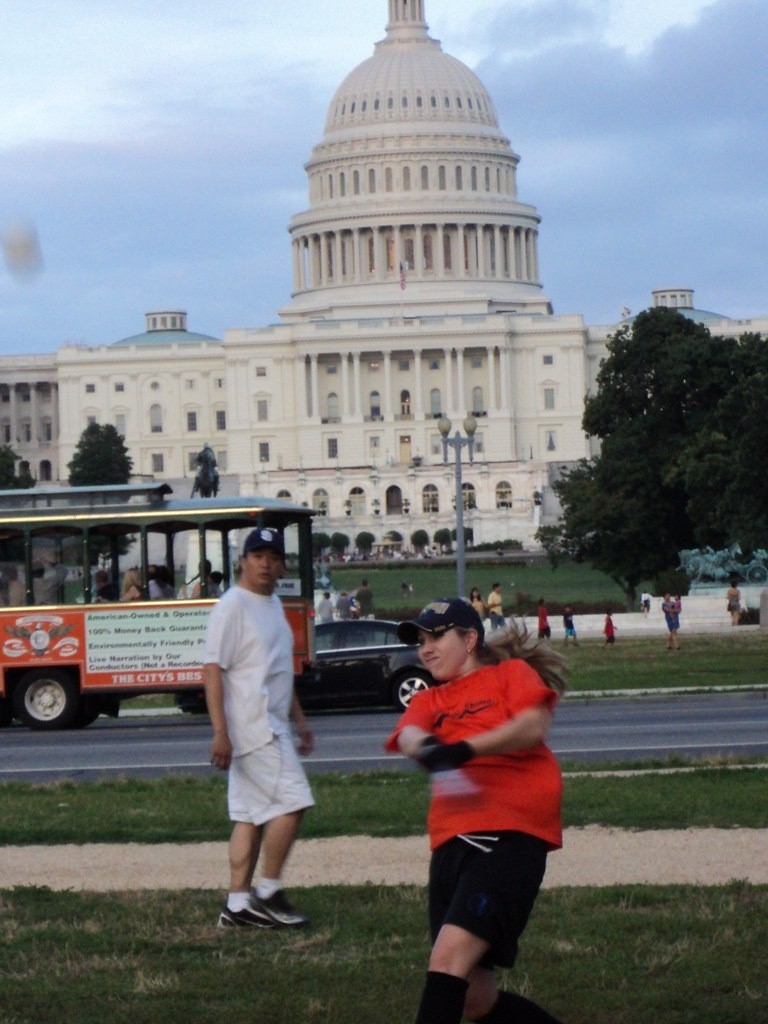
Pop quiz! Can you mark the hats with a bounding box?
[396,598,485,645]
[243,527,286,556]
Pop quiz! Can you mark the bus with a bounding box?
[1,483,323,731]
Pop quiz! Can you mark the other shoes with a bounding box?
[217,903,283,929]
[254,890,309,927]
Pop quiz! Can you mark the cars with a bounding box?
[297,620,445,712]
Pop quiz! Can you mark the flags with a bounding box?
[399,262,407,290]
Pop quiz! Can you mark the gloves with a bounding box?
[410,735,476,774]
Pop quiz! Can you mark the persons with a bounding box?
[538,599,550,640]
[670,593,681,624]
[641,590,651,620]
[726,580,741,627]
[487,583,505,631]
[563,605,579,648]
[603,609,616,648]
[200,528,315,926]
[662,592,679,651]
[470,587,488,620]
[311,545,448,621]
[384,598,572,1024]
[0,555,225,607]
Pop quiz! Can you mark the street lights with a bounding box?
[438,412,476,598]
